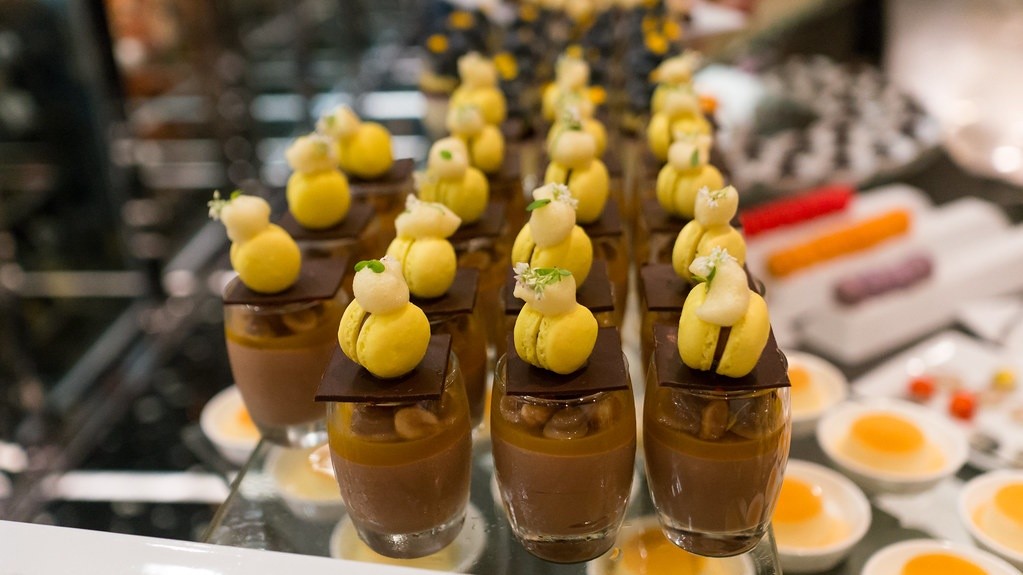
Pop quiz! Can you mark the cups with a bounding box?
[324,342,478,560]
[641,334,792,557]
[224,268,348,448]
[491,352,633,564]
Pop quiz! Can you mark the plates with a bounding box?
[958,467,1023,566]
[861,542,1023,575]
[813,395,969,494]
[328,496,490,575]
[586,516,756,575]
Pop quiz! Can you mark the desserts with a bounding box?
[213,1,799,558]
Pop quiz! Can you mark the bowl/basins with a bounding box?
[201,380,264,464]
[773,351,850,442]
[265,434,347,522]
[758,458,872,574]
